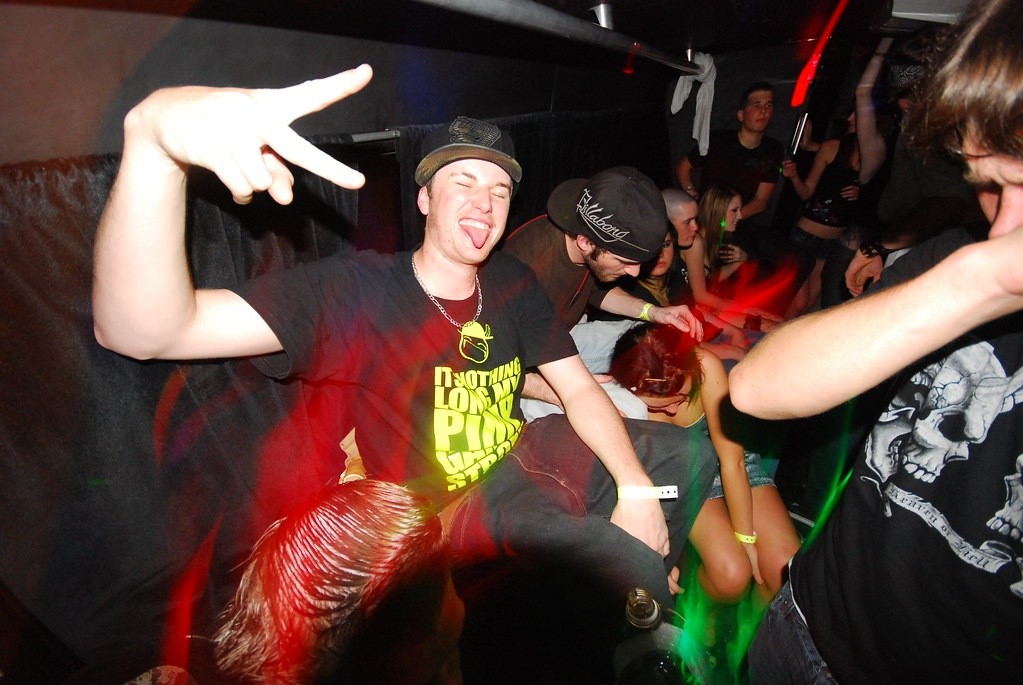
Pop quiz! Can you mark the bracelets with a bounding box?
[636,303,654,320]
[875,48,888,53]
[685,185,695,192]
[862,236,886,258]
[874,53,887,57]
[733,531,757,543]
[618,485,678,500]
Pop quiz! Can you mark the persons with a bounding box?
[92,37,975,685]
[728,0,1022,685]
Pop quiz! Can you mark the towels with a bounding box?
[670,51,717,157]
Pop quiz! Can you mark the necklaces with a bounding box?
[411,252,482,327]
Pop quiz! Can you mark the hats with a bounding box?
[415,116,522,187]
[546,166,667,262]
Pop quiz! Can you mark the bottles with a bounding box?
[613,586,714,685]
